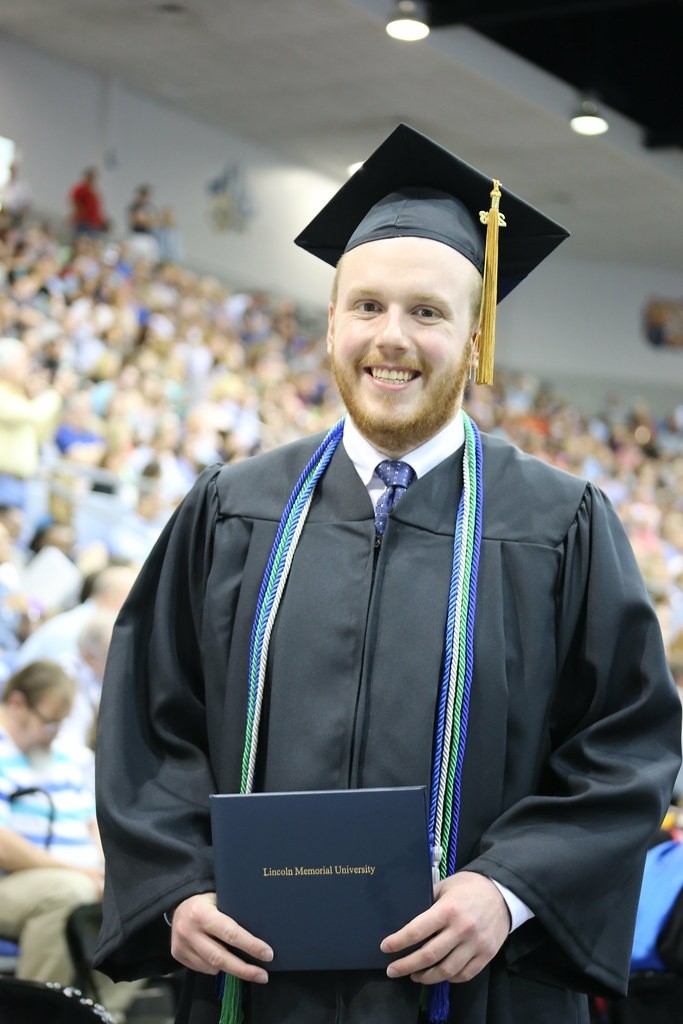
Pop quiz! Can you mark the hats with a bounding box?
[294,121,571,387]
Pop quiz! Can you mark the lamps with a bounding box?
[570,96,608,136]
[384,0,429,40]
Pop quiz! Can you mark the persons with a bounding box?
[0,163,683,1022]
[90,122,683,1023]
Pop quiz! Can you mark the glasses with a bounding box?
[26,703,59,732]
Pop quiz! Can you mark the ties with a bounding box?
[374,460,417,544]
